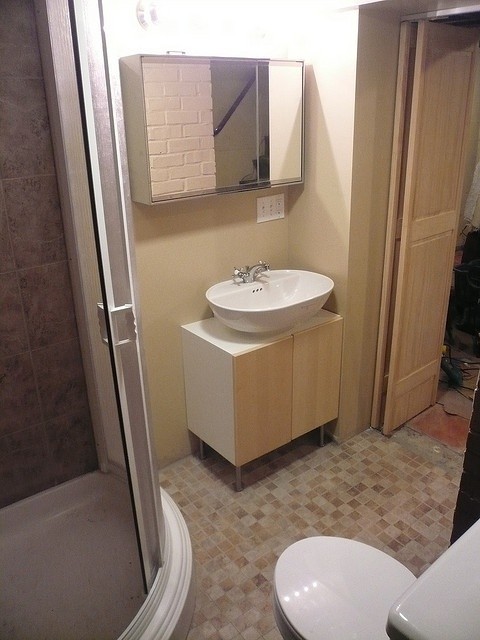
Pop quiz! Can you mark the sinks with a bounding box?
[206,270,334,334]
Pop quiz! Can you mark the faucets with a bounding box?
[246,264,269,279]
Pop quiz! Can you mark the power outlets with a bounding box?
[257,194,285,222]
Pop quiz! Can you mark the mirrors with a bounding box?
[140,55,303,203]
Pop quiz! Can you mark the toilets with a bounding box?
[274,519,480,640]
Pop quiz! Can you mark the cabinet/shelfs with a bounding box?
[180,308,344,492]
